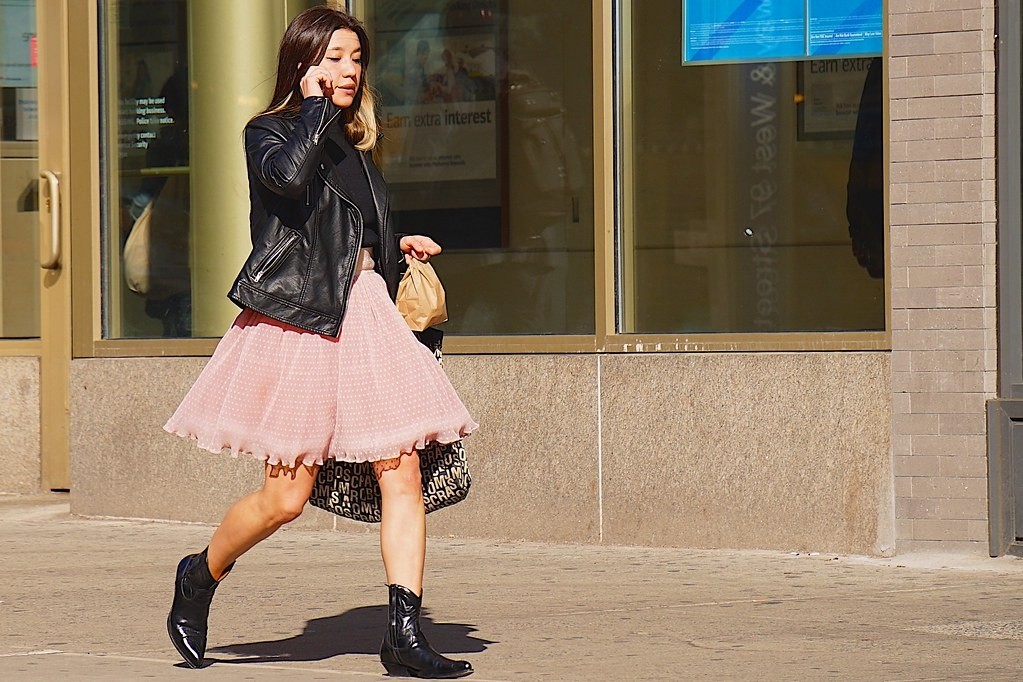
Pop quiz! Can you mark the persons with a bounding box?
[159,11,477,680]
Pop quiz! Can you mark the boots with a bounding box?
[381,584,472,680]
[168,551,237,668]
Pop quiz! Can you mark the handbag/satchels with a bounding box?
[123,159,189,301]
[396,254,447,332]
[307,329,470,523]
